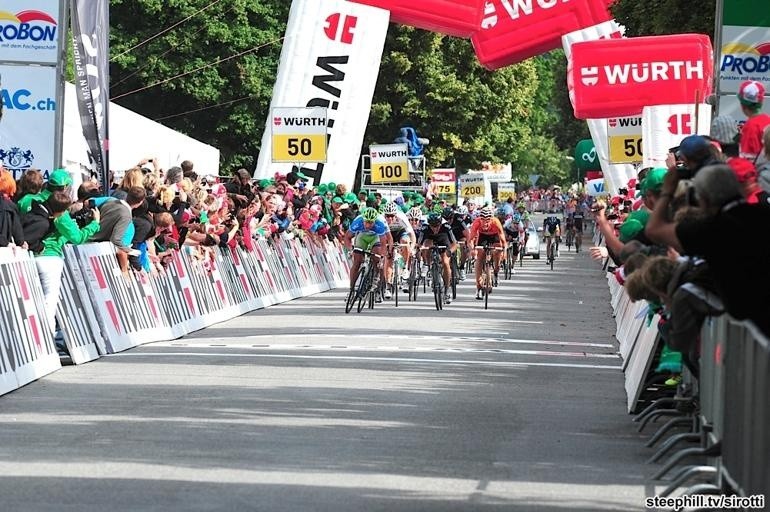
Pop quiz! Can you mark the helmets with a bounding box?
[427,213,442,226]
[383,201,397,215]
[511,214,521,224]
[517,203,526,209]
[457,205,468,216]
[362,207,378,222]
[407,207,423,221]
[478,207,493,220]
[442,207,455,219]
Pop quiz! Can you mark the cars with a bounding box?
[524,219,543,259]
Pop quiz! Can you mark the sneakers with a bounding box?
[344,267,500,304]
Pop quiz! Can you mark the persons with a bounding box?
[0,80,770,386]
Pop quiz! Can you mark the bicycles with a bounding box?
[543,235,562,270]
[566,228,582,253]
[345,234,525,314]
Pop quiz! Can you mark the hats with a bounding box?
[610,78,765,244]
[47,165,445,212]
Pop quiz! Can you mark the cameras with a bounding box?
[623,199,632,206]
[668,145,682,161]
[618,188,627,195]
[89,200,95,209]
[635,183,641,190]
[148,159,153,163]
[202,177,206,186]
[241,201,247,209]
[175,191,181,196]
